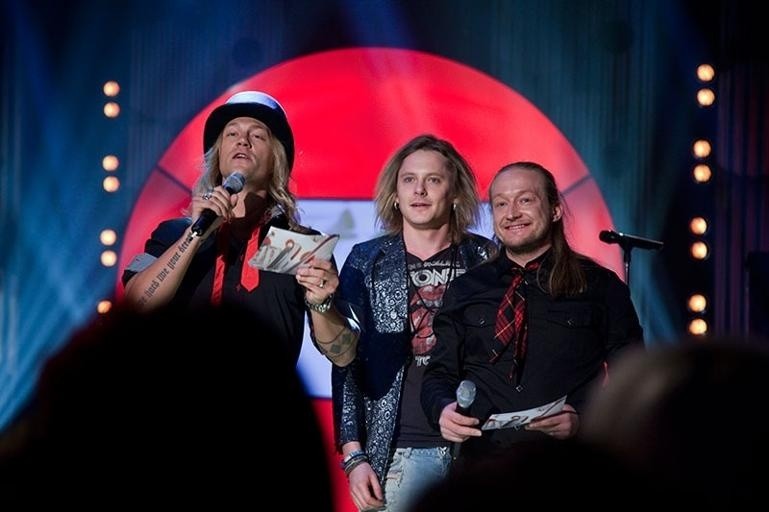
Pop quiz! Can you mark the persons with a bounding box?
[398,428,675,512]
[420,162,647,443]
[0,294,336,512]
[581,334,769,512]
[121,90,360,369]
[331,134,498,512]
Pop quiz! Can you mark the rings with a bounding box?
[203,195,210,199]
[320,279,324,288]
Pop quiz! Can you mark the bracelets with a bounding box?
[339,452,368,475]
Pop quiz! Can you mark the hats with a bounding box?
[203,90,294,173]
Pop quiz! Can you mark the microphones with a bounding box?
[452,379,477,460]
[188,172,245,240]
[598,229,662,251]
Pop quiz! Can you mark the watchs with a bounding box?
[304,296,335,313]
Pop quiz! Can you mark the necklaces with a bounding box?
[404,243,457,339]
